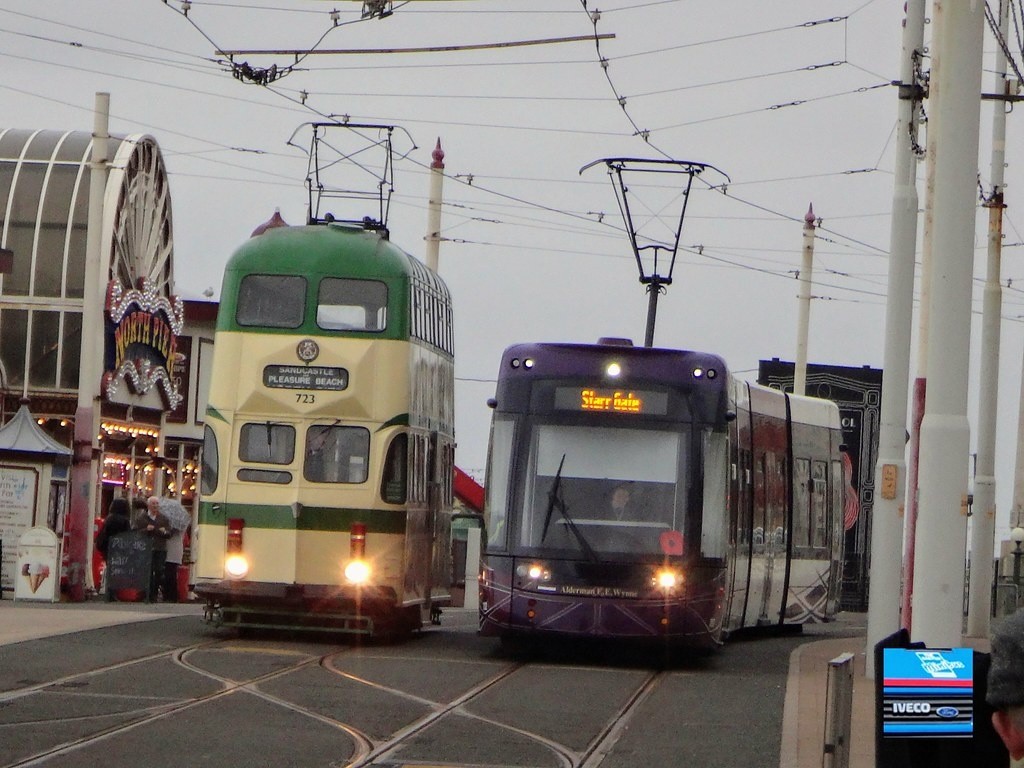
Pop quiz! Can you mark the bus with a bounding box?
[188,112,458,644]
[475,158,848,652]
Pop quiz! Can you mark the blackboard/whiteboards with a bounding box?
[106,530,153,593]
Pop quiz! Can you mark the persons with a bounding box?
[138,496,172,603]
[102,498,132,564]
[158,514,184,603]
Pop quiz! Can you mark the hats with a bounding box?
[985,603,1024,706]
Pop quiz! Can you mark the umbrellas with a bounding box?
[155,496,191,532]
